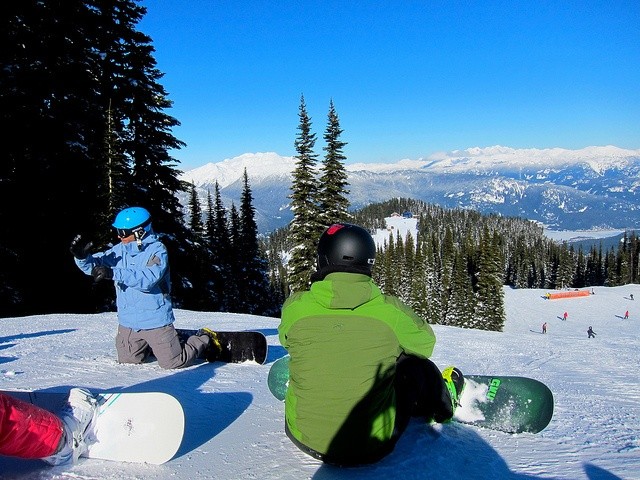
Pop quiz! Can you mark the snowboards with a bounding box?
[148,330,268,365]
[268,356,554,434]
[1,391,186,465]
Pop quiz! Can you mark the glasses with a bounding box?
[117,229,126,238]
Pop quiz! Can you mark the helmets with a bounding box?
[112,207,151,231]
[317,223,376,267]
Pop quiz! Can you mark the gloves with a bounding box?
[92,265,112,282]
[69,235,93,259]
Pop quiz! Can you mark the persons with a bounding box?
[0,385,96,466]
[624,310,629,319]
[562,312,568,321]
[277,223,465,467]
[542,321,547,333]
[587,326,597,339]
[631,293,635,300]
[71,206,222,369]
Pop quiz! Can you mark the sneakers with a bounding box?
[442,367,464,422]
[43,388,98,466]
[196,327,220,363]
